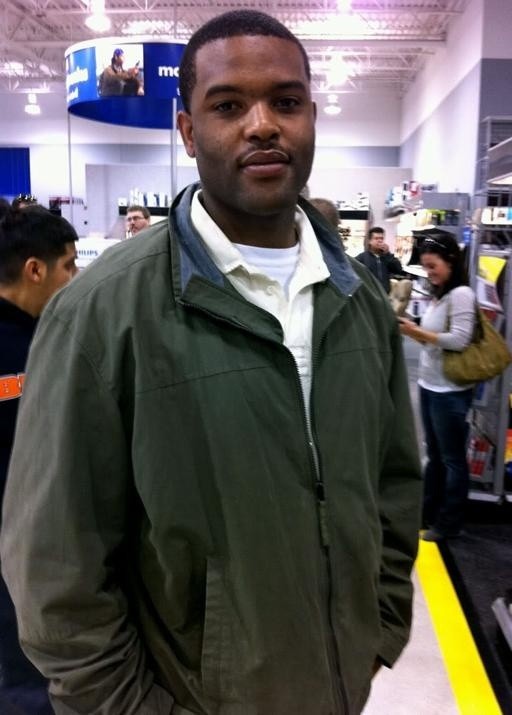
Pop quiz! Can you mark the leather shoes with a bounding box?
[422,527,446,542]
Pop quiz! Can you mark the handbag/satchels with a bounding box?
[441,287,511,387]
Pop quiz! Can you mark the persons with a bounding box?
[98,47,140,96]
[1,8,429,714]
[353,227,402,299]
[307,197,340,232]
[393,232,479,543]
[1,204,79,715]
[125,204,150,235]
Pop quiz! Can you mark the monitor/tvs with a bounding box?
[0,143,31,203]
